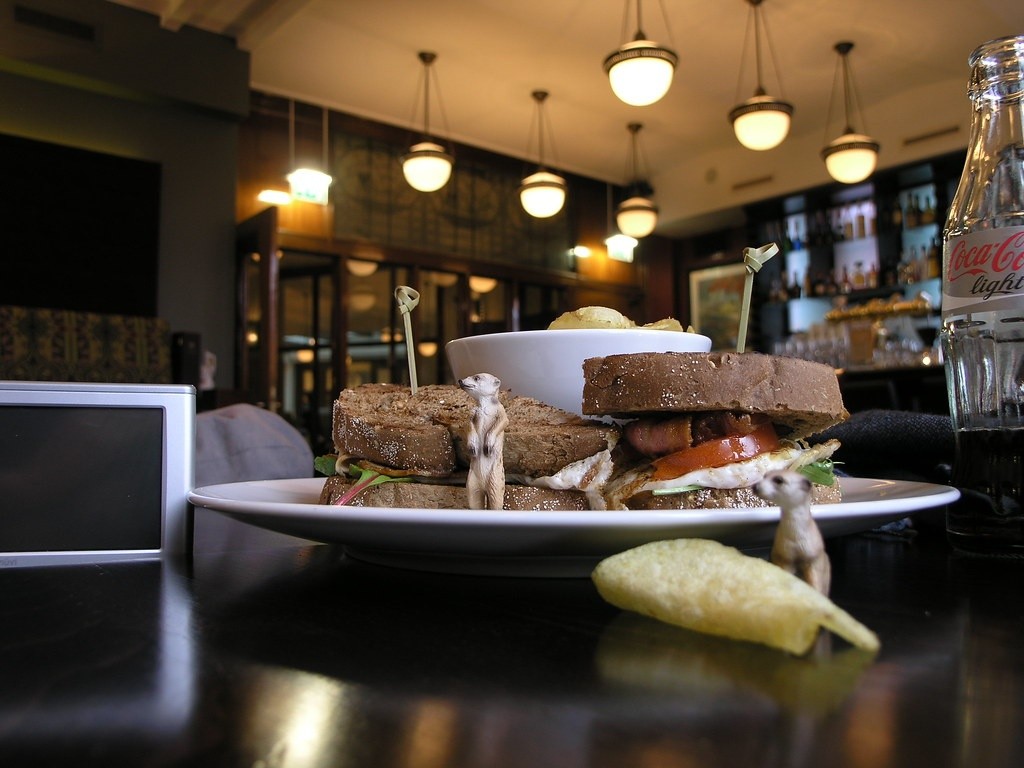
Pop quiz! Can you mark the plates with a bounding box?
[187,475,961,579]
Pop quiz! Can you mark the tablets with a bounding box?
[0,380,196,573]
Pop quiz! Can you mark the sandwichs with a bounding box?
[582,351,849,512]
[321,383,613,513]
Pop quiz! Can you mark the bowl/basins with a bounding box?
[446,329,712,427]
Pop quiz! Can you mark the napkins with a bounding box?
[801,408,1024,536]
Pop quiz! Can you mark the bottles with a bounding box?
[764,262,879,302]
[890,192,935,227]
[896,238,939,283]
[814,199,874,238]
[762,219,805,252]
[941,37,1024,553]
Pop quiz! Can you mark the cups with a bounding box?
[768,328,850,370]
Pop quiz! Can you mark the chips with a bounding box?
[594,540,879,656]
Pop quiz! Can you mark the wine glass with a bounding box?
[878,313,940,367]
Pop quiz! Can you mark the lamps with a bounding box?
[615,123,658,238]
[819,42,878,185]
[514,91,569,218]
[602,0,679,107]
[398,52,456,193]
[729,1,795,150]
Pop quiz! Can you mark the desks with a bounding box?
[0,526,1024,768]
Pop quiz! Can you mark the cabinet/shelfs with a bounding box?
[743,149,969,350]
